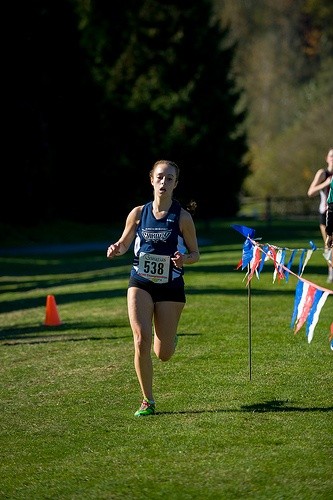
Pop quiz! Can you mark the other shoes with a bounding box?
[326,271,333,283]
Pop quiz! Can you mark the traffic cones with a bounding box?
[42,295,63,327]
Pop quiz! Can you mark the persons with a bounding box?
[308,150,333,284]
[107,161,200,417]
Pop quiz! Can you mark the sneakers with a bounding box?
[133,398,155,416]
